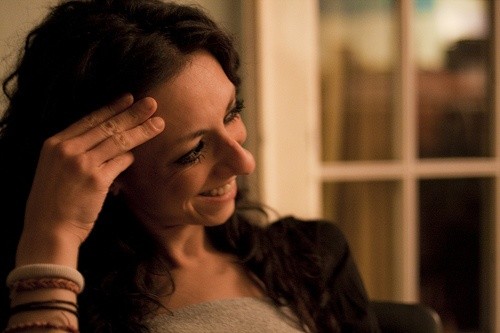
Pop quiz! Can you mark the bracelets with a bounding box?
[5,263,85,333]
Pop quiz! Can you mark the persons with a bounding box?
[0,0,381,333]
